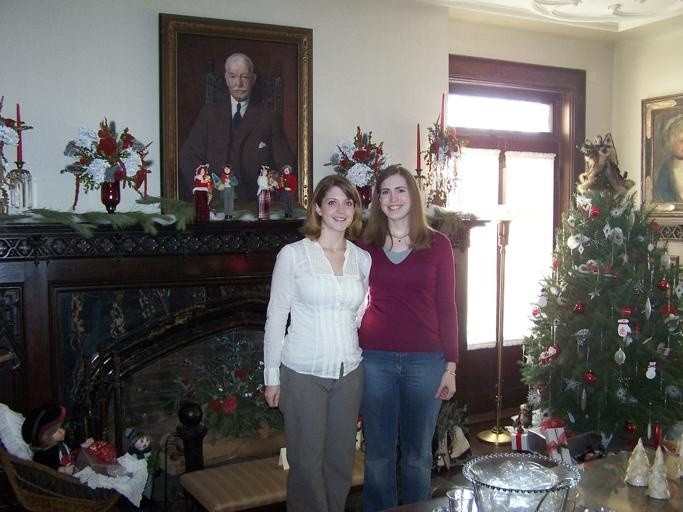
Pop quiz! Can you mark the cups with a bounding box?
[446,487,474,512]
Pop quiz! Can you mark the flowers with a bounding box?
[0,95,33,191]
[172,327,278,439]
[59,114,155,210]
[418,122,464,208]
[323,125,401,189]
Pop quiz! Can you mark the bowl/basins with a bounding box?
[462,452,580,512]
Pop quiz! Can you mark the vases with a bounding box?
[0,188,8,215]
[356,184,372,209]
[101,181,120,214]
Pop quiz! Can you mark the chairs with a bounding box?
[0,401,123,512]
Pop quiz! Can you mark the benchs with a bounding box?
[178,449,365,512]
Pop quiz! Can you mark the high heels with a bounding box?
[434,426,472,473]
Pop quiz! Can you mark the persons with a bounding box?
[279,165,298,218]
[656,114,683,204]
[357,163,460,512]
[257,166,274,220]
[22,405,94,475]
[263,174,372,512]
[218,165,238,219]
[193,164,211,223]
[117,427,153,473]
[179,53,298,202]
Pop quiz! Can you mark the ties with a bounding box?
[232,103,242,125]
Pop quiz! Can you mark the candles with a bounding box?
[441,90,446,132]
[417,123,421,169]
[16,103,22,162]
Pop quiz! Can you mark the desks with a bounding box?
[375,447,683,511]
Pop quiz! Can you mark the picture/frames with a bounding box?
[157,12,314,215]
[639,93,683,217]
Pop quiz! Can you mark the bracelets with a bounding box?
[446,370,457,376]
